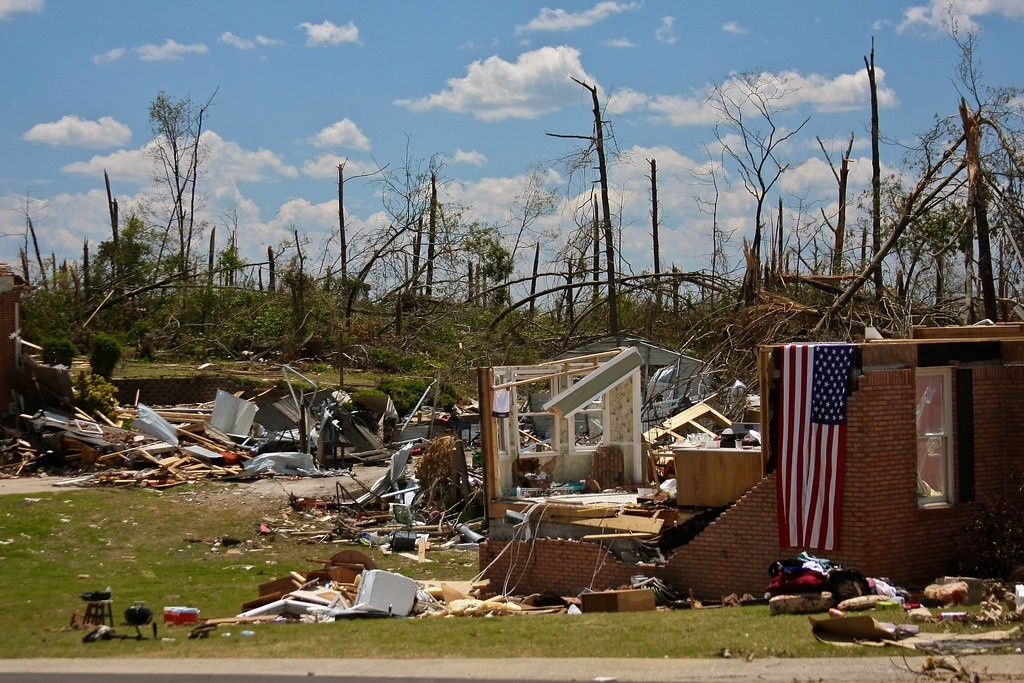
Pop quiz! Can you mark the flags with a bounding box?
[778,342,853,550]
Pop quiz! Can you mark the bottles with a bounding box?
[106,586,111,601]
[242,631,256,636]
